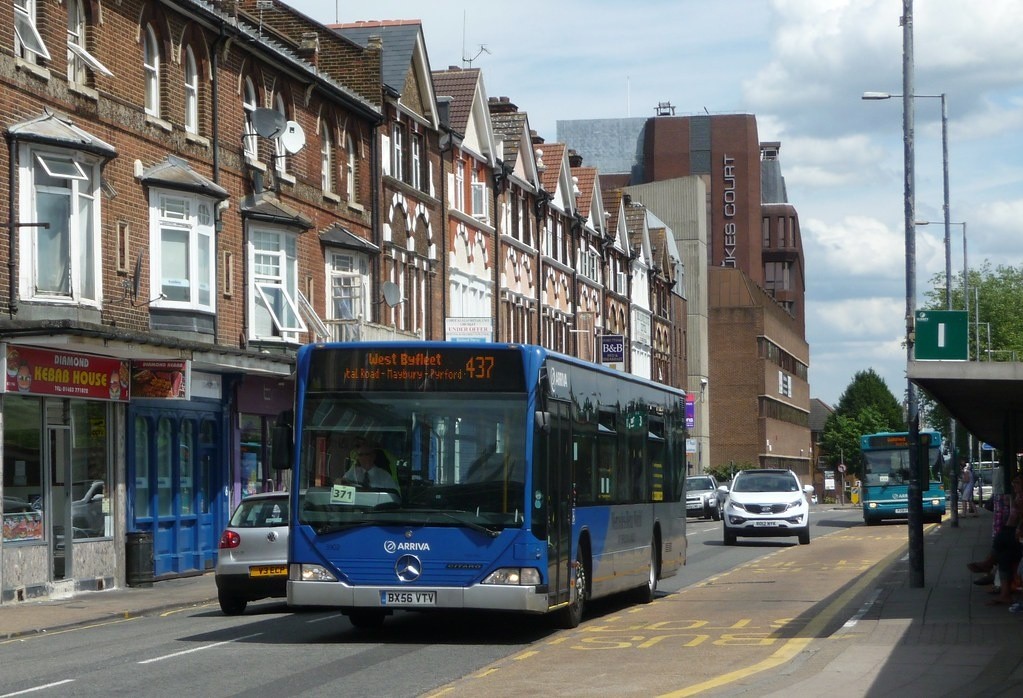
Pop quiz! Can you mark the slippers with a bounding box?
[971,515,978,518]
[958,514,965,518]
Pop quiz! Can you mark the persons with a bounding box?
[958,458,980,519]
[333,440,402,498]
[967,458,1023,614]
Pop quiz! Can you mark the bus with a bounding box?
[282,339,690,630]
[859,428,946,525]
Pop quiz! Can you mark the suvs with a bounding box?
[686,474,719,520]
[723,469,814,548]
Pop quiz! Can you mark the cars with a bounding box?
[32,478,106,576]
[213,489,336,616]
[3,494,41,525]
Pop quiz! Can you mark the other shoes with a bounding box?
[974,574,995,585]
[970,560,992,574]
[988,597,1013,604]
[1009,602,1023,612]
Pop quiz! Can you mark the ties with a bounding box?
[362,472,370,488]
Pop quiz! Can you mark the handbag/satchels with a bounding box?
[985,494,995,512]
[958,480,963,490]
[973,472,978,484]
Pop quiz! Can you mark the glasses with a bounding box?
[357,451,371,458]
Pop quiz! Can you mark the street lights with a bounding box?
[861,91,959,528]
[914,220,970,311]
